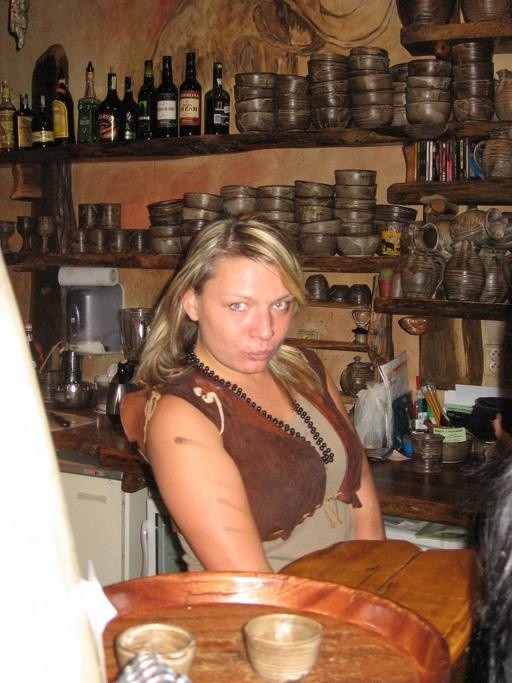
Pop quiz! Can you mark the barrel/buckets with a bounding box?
[94,375,113,412]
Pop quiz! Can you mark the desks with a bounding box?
[270,531,483,683]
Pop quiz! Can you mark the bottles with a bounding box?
[1,50,231,151]
[24,321,39,373]
[390,272,403,299]
[493,69,511,122]
[340,356,374,397]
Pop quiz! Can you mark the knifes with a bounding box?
[46,410,69,427]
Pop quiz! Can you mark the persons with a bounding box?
[0,247,110,683]
[490,409,511,443]
[127,214,389,574]
[459,448,511,681]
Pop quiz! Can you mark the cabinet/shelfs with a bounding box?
[0,119,414,390]
[366,10,511,329]
[365,440,512,681]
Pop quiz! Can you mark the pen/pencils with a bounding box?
[421,384,441,427]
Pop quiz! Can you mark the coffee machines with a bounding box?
[106,306,161,424]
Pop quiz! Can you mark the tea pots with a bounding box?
[400,207,512,304]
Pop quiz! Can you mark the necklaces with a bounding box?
[184,346,337,465]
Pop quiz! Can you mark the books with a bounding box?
[375,349,409,449]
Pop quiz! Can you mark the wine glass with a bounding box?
[1,215,54,255]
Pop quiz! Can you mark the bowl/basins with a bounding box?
[145,168,417,258]
[401,431,472,463]
[53,382,95,410]
[235,40,495,133]
[395,0,511,27]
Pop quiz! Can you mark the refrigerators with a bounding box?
[59,467,149,595]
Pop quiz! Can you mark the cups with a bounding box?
[304,273,373,305]
[67,201,149,254]
[472,137,511,178]
[115,622,197,680]
[40,369,59,404]
[242,613,324,680]
[410,432,444,472]
